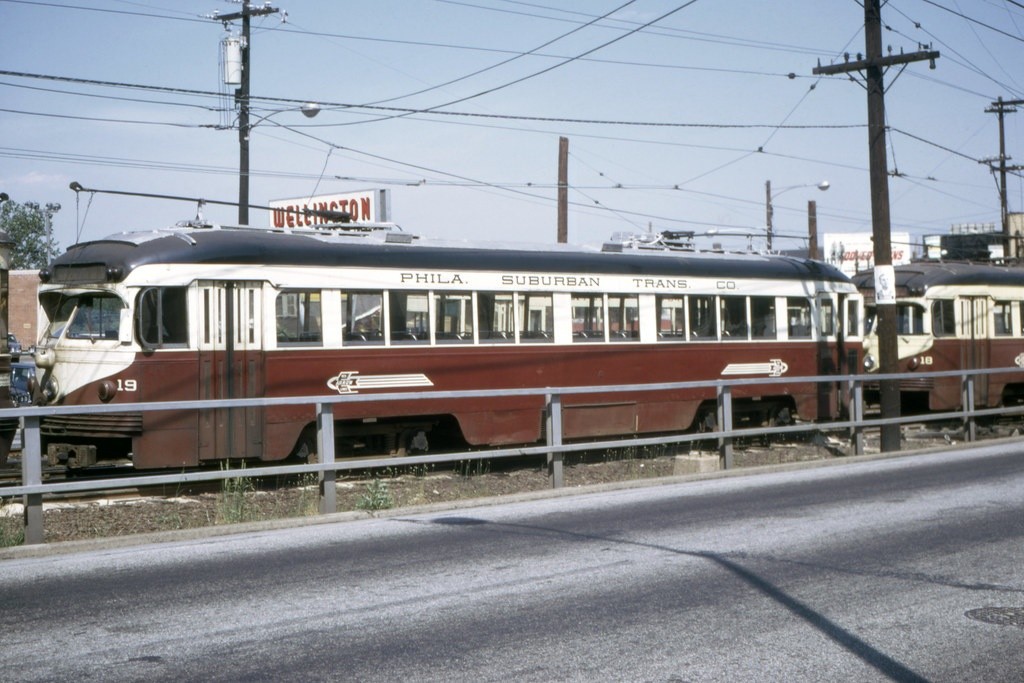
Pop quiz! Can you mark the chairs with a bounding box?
[349,330,733,340]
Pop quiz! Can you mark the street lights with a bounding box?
[233,102,325,228]
[761,178,831,252]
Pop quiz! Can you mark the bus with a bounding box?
[27,180,869,469]
[0,255,20,471]
[847,242,1024,412]
